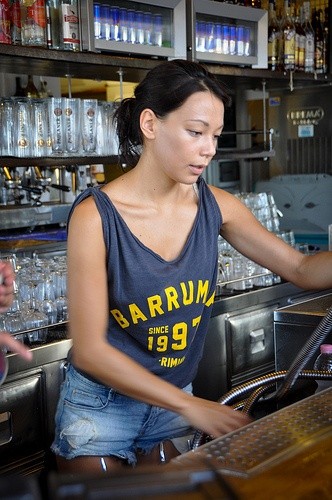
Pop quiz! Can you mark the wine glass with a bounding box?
[0,250,69,353]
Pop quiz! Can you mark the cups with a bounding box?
[277,232,310,255]
[0,97,122,158]
[235,191,281,232]
[215,234,281,290]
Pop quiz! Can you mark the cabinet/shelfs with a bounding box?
[0,44,332,232]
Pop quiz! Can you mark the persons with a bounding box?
[51,59,332,481]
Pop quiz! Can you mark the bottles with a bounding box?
[24,75,39,99]
[303,1,315,72]
[311,8,332,74]
[296,0,305,72]
[0,0,11,44]
[313,344,332,394]
[46,0,60,51]
[276,0,296,71]
[11,0,22,46]
[39,76,49,99]
[268,0,279,71]
[20,0,47,47]
[11,77,27,104]
[59,0,80,52]
